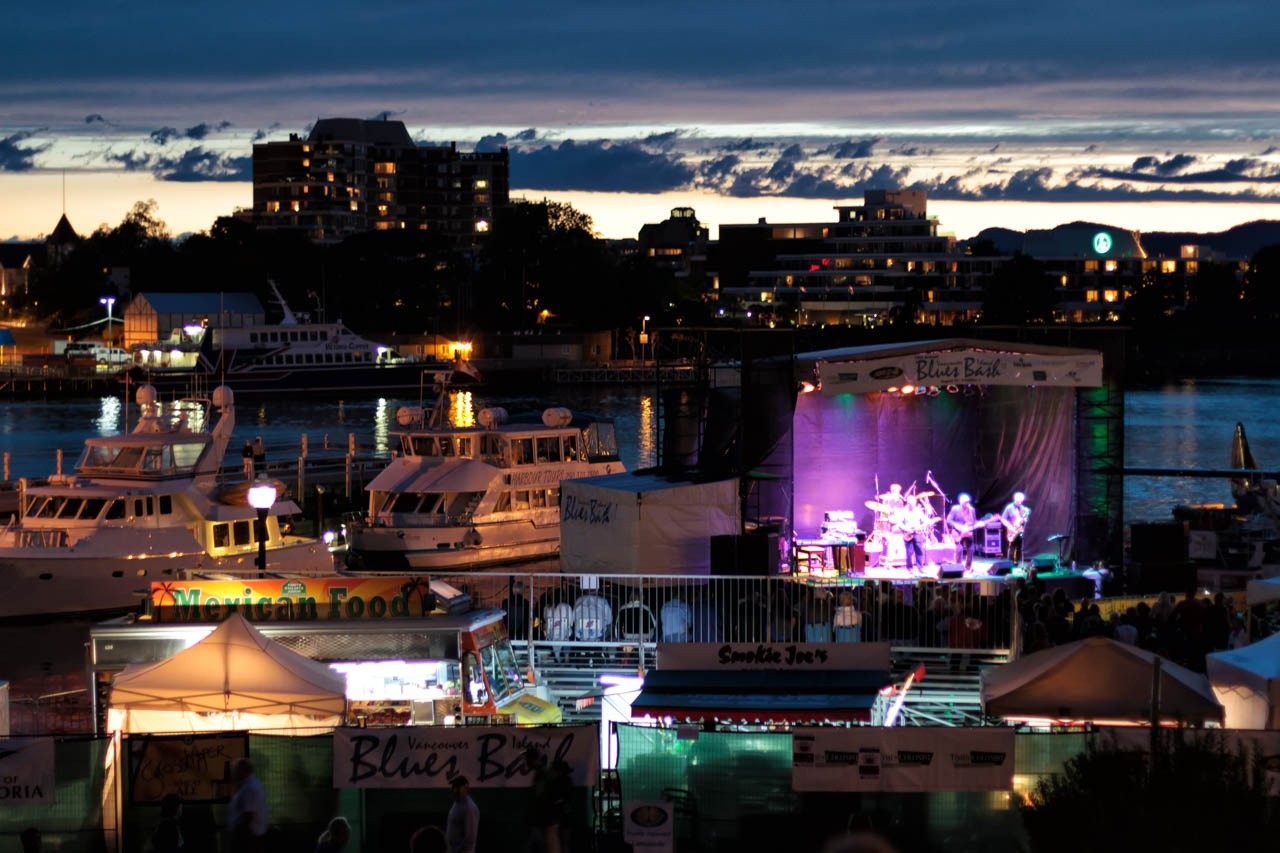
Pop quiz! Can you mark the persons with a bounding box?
[882,484,906,521]
[1001,492,1029,565]
[152,793,185,853]
[1002,569,1280,675]
[241,440,254,480]
[1175,534,1280,598]
[20,828,43,853]
[895,495,930,575]
[947,494,985,574]
[223,757,352,853]
[408,775,593,853]
[253,437,265,478]
[502,580,724,644]
[736,582,1034,664]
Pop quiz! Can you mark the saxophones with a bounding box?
[1007,503,1032,542]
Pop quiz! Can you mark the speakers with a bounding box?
[938,564,965,578]
[1032,557,1056,573]
[991,562,1013,575]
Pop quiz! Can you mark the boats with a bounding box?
[1,300,384,619]
[335,370,631,573]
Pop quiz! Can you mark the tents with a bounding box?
[1245,578,1280,644]
[788,337,1102,582]
[983,637,1226,730]
[103,609,368,853]
[561,464,742,588]
[1206,631,1280,730]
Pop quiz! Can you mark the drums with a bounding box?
[874,519,891,536]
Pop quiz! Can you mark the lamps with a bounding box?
[928,386,941,396]
[946,384,959,393]
[963,384,977,397]
[916,386,927,395]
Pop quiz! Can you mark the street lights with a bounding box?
[100,298,115,372]
[795,308,805,326]
[641,316,650,368]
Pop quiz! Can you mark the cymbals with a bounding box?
[915,491,934,499]
[880,494,900,499]
[865,501,883,511]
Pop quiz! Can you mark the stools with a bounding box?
[799,548,825,574]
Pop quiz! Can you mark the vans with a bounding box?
[96,346,133,365]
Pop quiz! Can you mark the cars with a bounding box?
[375,351,407,365]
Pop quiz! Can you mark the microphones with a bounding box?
[926,471,930,483]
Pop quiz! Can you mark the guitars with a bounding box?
[900,516,941,542]
[951,513,1000,542]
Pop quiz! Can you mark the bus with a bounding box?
[66,341,103,357]
